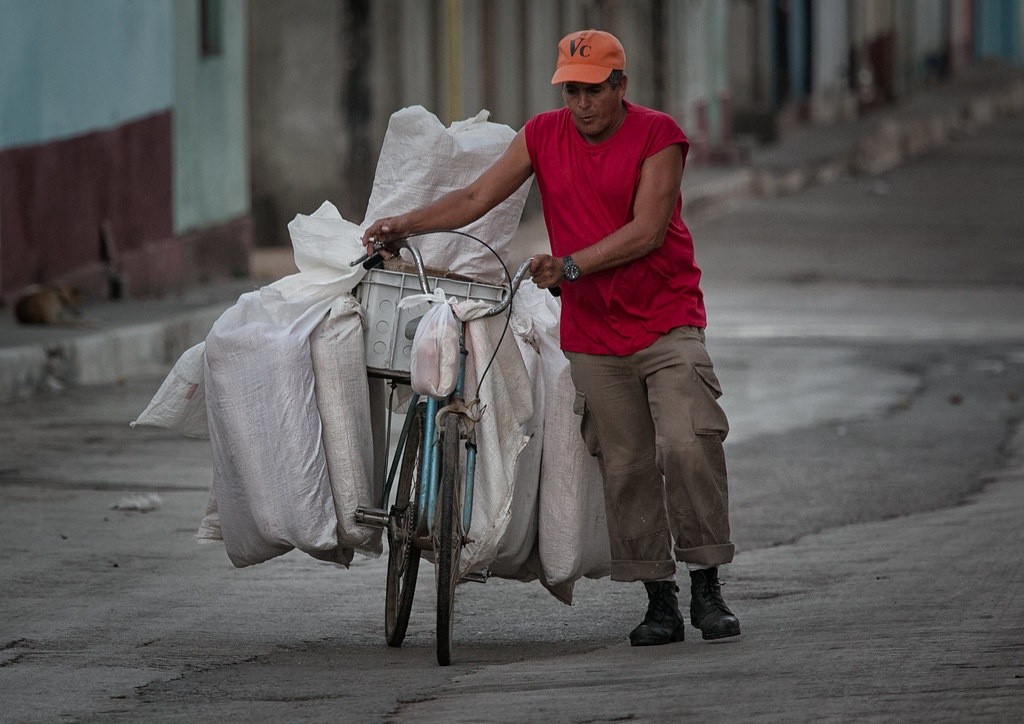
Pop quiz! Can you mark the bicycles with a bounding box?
[348,230,562,667]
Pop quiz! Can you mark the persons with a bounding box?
[361,28,741,647]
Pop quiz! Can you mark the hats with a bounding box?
[550,28,626,86]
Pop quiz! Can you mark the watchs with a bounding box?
[562,256,580,279]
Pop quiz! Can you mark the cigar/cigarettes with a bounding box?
[360,236,375,242]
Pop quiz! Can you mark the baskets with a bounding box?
[356,268,504,378]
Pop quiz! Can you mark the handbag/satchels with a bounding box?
[397,287,462,397]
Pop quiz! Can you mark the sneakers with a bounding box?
[690,578,741,640]
[628,584,685,646]
